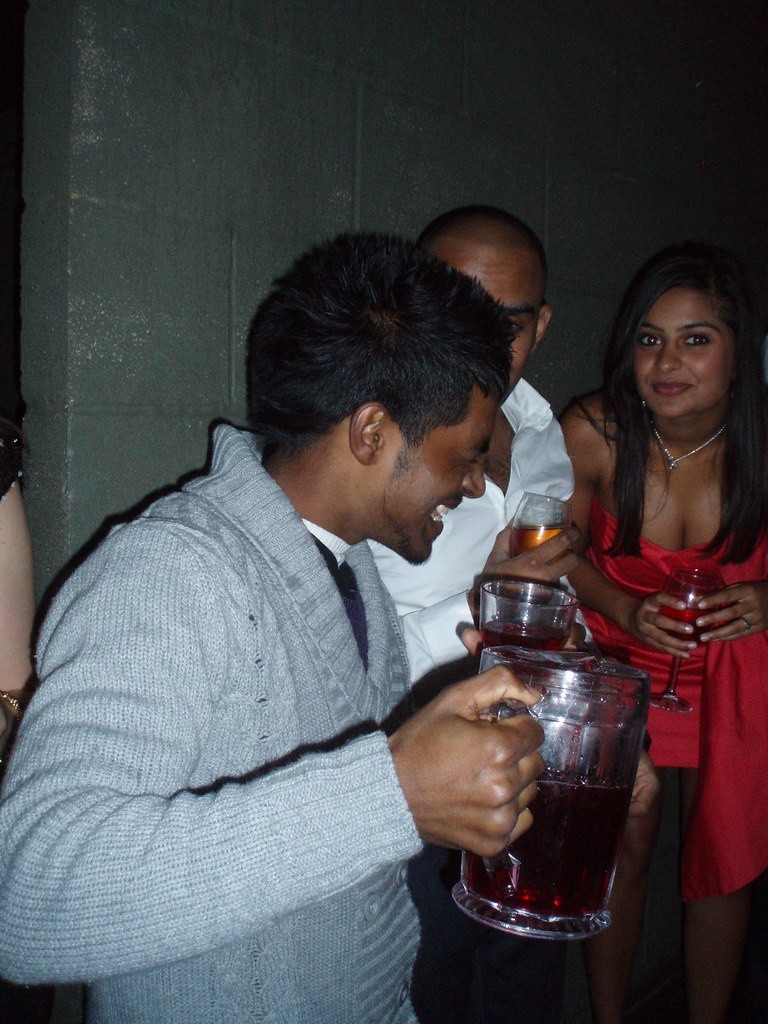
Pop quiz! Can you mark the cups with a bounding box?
[509,492,573,565]
[477,581,580,651]
[452,645,651,940]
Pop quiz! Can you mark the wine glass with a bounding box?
[651,566,722,711]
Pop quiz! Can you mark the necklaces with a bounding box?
[649,415,732,470]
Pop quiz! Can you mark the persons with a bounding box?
[368,205,594,1024]
[0,232,542,1024]
[560,240,768,1024]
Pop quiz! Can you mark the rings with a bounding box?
[741,617,752,631]
